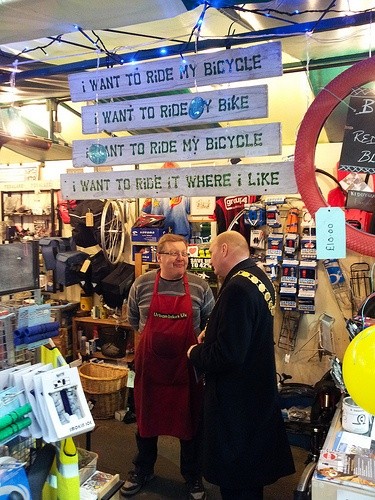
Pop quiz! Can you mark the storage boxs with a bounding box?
[132,219,168,241]
[242,196,318,319]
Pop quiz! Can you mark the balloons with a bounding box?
[342,323,375,416]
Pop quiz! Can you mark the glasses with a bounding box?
[159,250,188,257]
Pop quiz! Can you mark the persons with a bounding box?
[120,234,216,500]
[187,231,297,499]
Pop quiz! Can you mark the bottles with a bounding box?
[122,299,127,319]
[39,271,46,287]
[80,332,86,352]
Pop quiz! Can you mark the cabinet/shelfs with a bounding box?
[0,189,62,246]
[71,253,148,409]
[130,214,225,290]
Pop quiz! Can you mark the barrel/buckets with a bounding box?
[342,397,370,434]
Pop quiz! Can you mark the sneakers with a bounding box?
[185,477,207,500]
[120,471,154,497]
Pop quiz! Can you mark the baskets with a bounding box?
[79,363,128,393]
[83,386,127,419]
